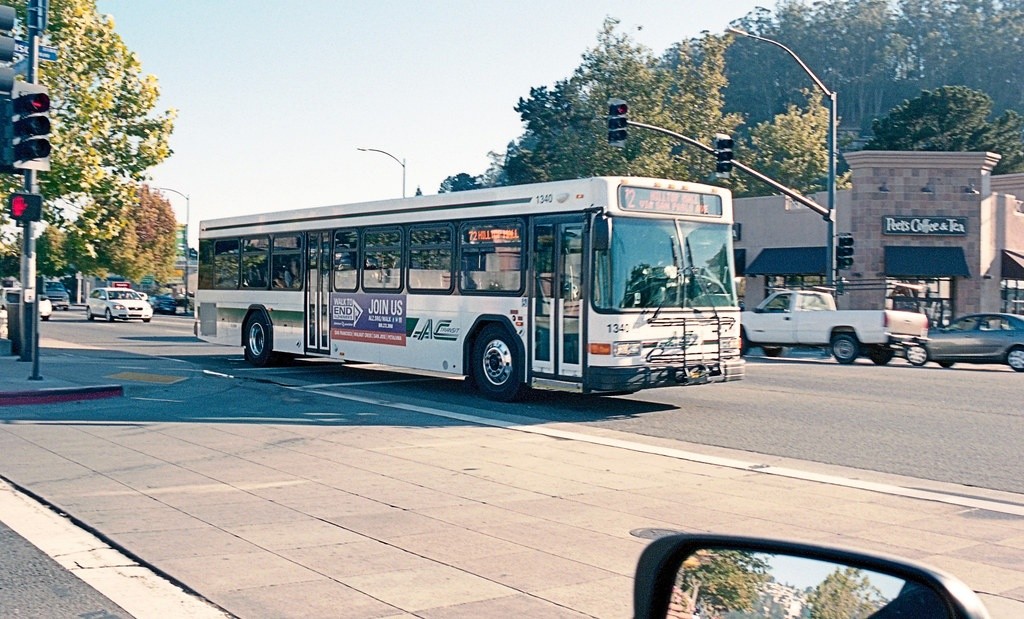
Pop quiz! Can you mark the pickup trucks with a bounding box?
[737,290,929,364]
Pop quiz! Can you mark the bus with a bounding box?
[194,178,744,399]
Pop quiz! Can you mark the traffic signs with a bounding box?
[12,37,58,61]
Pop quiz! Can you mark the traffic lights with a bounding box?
[7,192,43,224]
[0,2,17,91]
[603,97,628,147]
[714,131,733,176]
[835,231,855,271]
[8,80,53,173]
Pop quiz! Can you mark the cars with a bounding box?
[148,295,176,314]
[84,287,154,323]
[902,309,1024,372]
[42,281,69,310]
[0,288,52,321]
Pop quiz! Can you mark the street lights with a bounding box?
[357,146,408,197]
[153,187,190,243]
[724,26,839,294]
